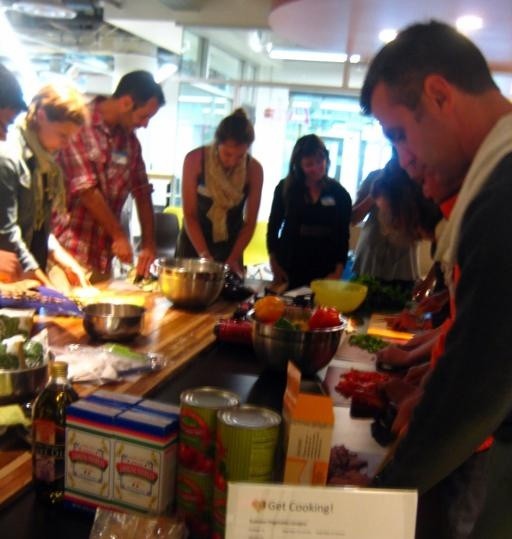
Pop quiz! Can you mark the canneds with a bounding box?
[213,406,281,539]
[175,387,240,533]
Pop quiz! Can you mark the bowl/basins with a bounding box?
[154,256,232,309]
[312,280,368,315]
[247,307,346,378]
[81,303,146,341]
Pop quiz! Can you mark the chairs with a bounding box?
[136,212,180,260]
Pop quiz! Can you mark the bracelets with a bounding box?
[198,249,209,258]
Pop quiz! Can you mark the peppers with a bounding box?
[255,296,341,331]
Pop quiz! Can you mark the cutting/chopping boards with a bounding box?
[366,313,413,341]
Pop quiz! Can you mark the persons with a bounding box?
[267,134,351,289]
[47,70,165,285]
[0,83,90,289]
[174,108,263,279]
[331,21,512,538]
[0,63,28,141]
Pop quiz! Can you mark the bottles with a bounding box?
[32,360,80,482]
